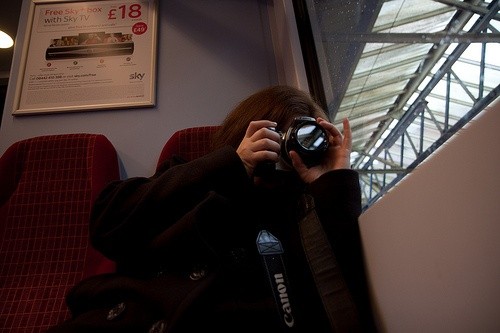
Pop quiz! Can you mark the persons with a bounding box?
[45,84,380,333]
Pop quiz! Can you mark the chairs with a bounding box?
[156,126,227,174]
[0,133,122,333]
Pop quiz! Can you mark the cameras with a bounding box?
[256,116,330,187]
